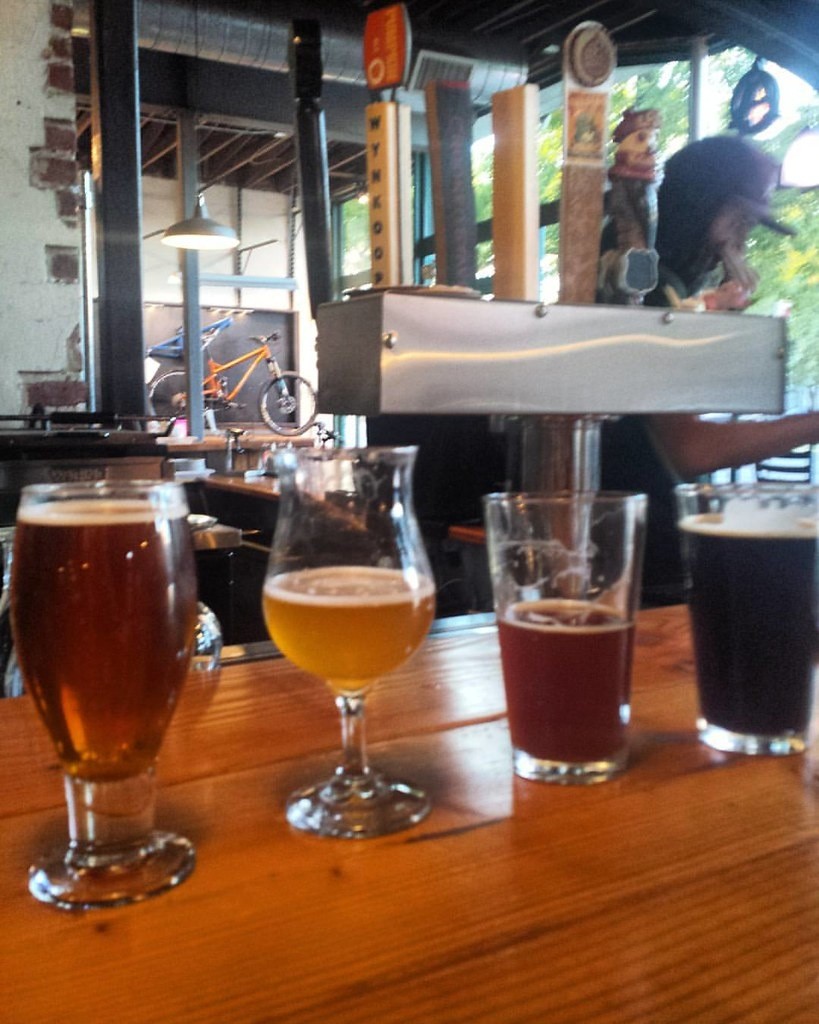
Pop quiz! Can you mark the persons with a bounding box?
[405,136,819,619]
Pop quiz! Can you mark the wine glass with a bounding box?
[264,445,437,840]
[13,482,197,905]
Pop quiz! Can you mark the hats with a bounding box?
[665,136,799,236]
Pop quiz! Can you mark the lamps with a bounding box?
[159,0,242,250]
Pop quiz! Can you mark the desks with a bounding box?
[169,434,315,473]
[0,605,818,1024]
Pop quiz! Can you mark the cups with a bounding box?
[484,492,640,785]
[675,482,818,757]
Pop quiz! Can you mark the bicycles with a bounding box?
[149,330,318,438]
